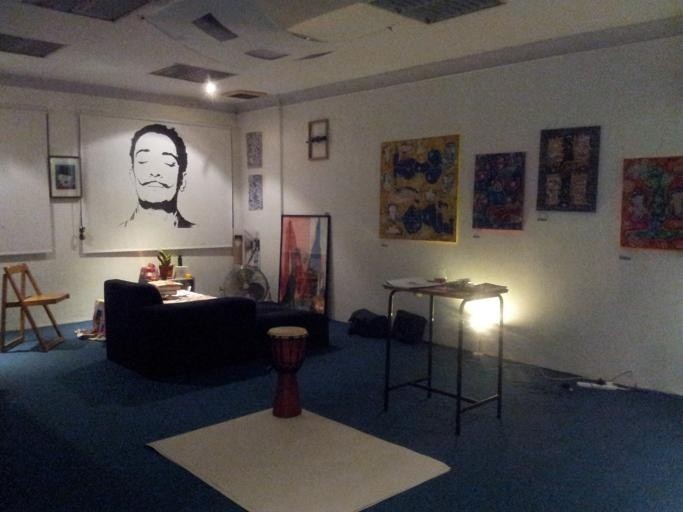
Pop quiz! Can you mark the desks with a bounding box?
[383,283,508,435]
[162,289,219,305]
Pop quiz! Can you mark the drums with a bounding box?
[268,327,307,418]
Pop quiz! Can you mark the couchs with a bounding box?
[103,278,261,377]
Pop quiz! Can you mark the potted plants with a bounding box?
[156,250,172,280]
[174,255,187,278]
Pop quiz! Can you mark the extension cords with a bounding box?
[576,380,617,391]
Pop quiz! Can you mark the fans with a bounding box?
[222,264,268,305]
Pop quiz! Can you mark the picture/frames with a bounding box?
[536,126,600,213]
[277,213,331,345]
[46,155,82,199]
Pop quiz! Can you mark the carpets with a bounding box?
[144,402,450,511]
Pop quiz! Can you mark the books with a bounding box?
[147,279,183,299]
[381,273,510,298]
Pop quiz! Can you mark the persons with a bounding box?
[109,124,197,232]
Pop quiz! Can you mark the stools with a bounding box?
[91,299,105,334]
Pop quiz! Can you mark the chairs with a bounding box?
[0,264,71,353]
